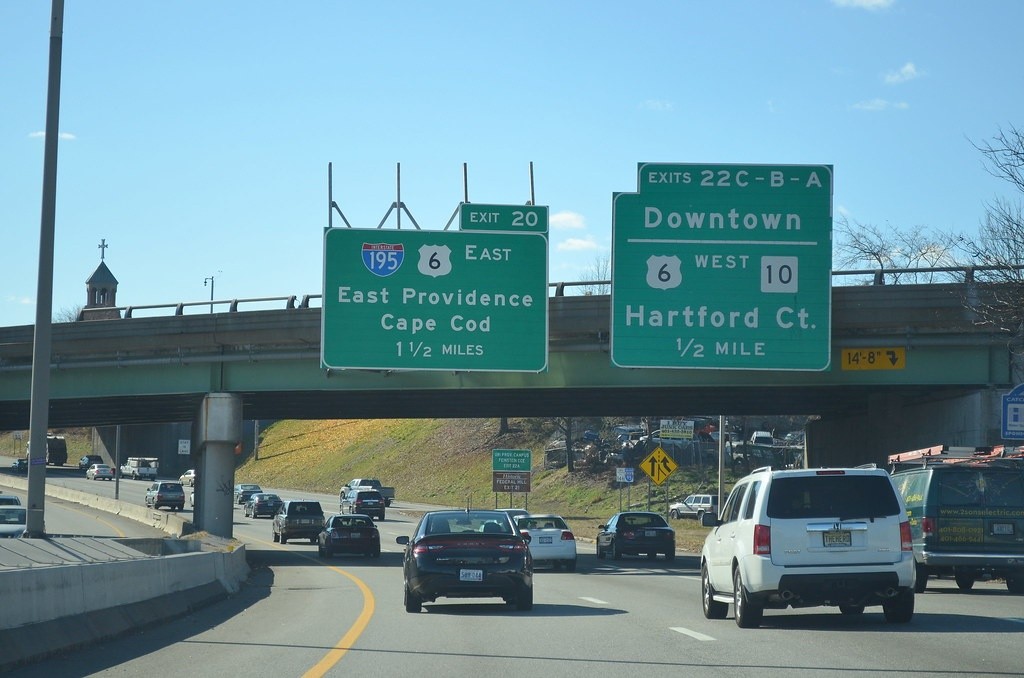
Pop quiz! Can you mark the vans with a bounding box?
[886,461,1024,594]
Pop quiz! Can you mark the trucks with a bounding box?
[26,433,68,465]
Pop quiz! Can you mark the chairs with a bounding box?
[356,522,366,526]
[520,521,529,529]
[335,521,343,527]
[544,523,553,528]
[483,522,501,533]
[431,517,450,534]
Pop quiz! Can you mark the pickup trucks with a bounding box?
[119,456,159,481]
[340,478,395,507]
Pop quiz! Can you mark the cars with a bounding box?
[189,490,195,507]
[78,455,114,480]
[11,458,28,477]
[608,416,774,453]
[395,508,576,614]
[0,495,27,534]
[596,512,675,562]
[178,469,196,487]
[234,484,285,519]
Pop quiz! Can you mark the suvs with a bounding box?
[670,494,729,520]
[339,489,386,520]
[272,500,327,544]
[145,481,185,512]
[700,463,917,628]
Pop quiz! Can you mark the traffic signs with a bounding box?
[611,162,835,371]
[319,228,548,374]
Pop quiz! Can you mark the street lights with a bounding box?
[204,276,214,314]
